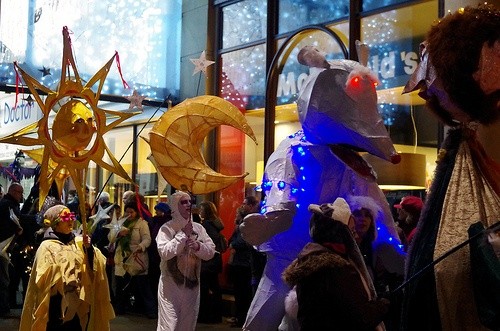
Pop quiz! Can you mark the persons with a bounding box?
[349,196,377,282]
[0,182,200,318]
[19,205,111,331]
[394,196,424,247]
[227,197,267,326]
[282,203,391,331]
[467,221,500,331]
[156,191,216,331]
[197,200,227,324]
